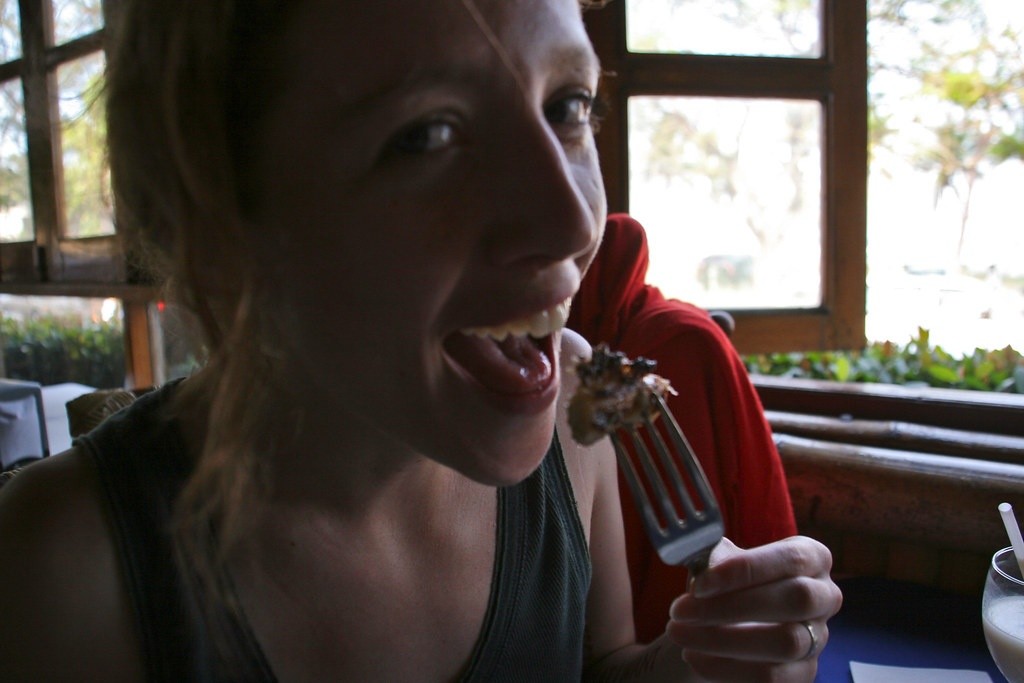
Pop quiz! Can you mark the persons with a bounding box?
[575,216,799,648]
[0,0,842,683]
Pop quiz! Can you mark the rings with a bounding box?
[801,621,818,659]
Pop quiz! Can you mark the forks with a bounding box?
[610,391,725,578]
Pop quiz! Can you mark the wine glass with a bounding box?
[981,545,1024,683]
